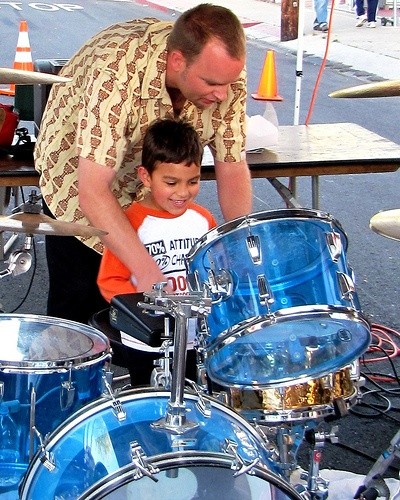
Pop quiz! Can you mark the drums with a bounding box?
[227,356,365,426]
[17,383,312,500]
[0,312,113,500]
[182,207,366,388]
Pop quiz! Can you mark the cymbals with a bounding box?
[0,67,73,85]
[368,209,400,241]
[327,80,400,99]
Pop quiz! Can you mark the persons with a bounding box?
[95,118,234,407]
[355,0,379,28]
[33,1,254,324]
[313,0,328,32]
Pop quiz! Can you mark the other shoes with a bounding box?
[318,22,328,32]
[314,20,319,30]
[355,14,368,27]
[366,21,376,28]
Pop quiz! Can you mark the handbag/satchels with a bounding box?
[0,102,21,148]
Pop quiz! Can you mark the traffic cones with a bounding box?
[251,49,284,102]
[0,20,35,96]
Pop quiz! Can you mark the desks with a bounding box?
[0,123,400,261]
